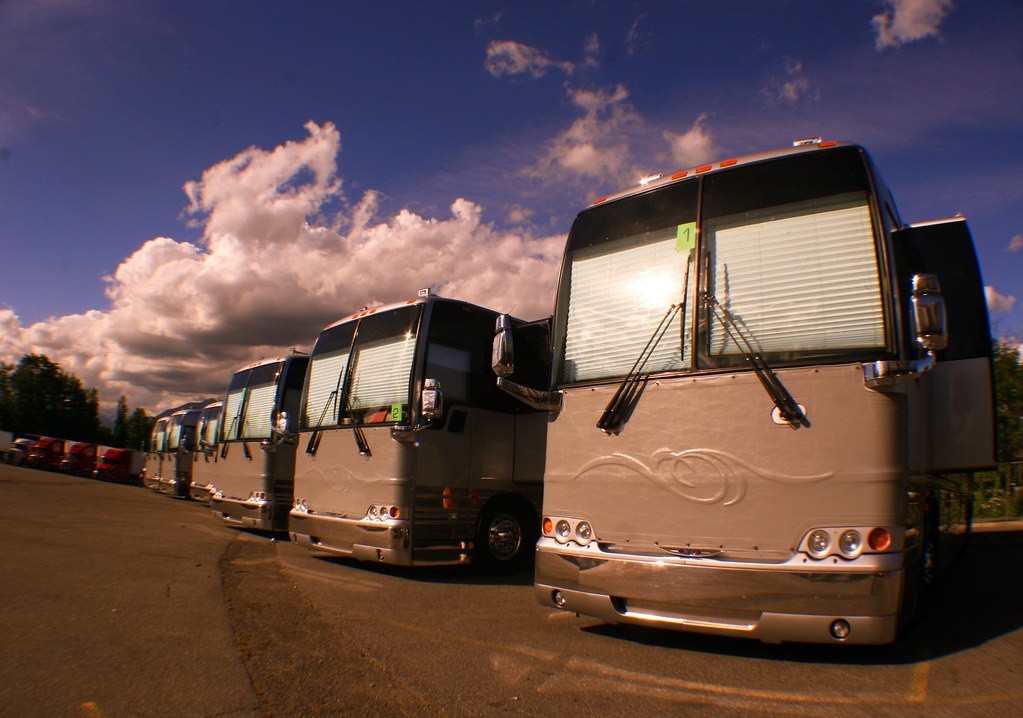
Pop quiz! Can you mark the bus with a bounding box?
[287,292,551,578]
[493,136,997,655]
[189,400,223,504]
[142,411,170,496]
[158,402,211,502]
[210,348,311,536]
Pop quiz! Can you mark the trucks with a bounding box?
[59,440,95,477]
[93,446,142,484]
[4,435,38,467]
[26,436,63,471]
[0,431,13,460]
